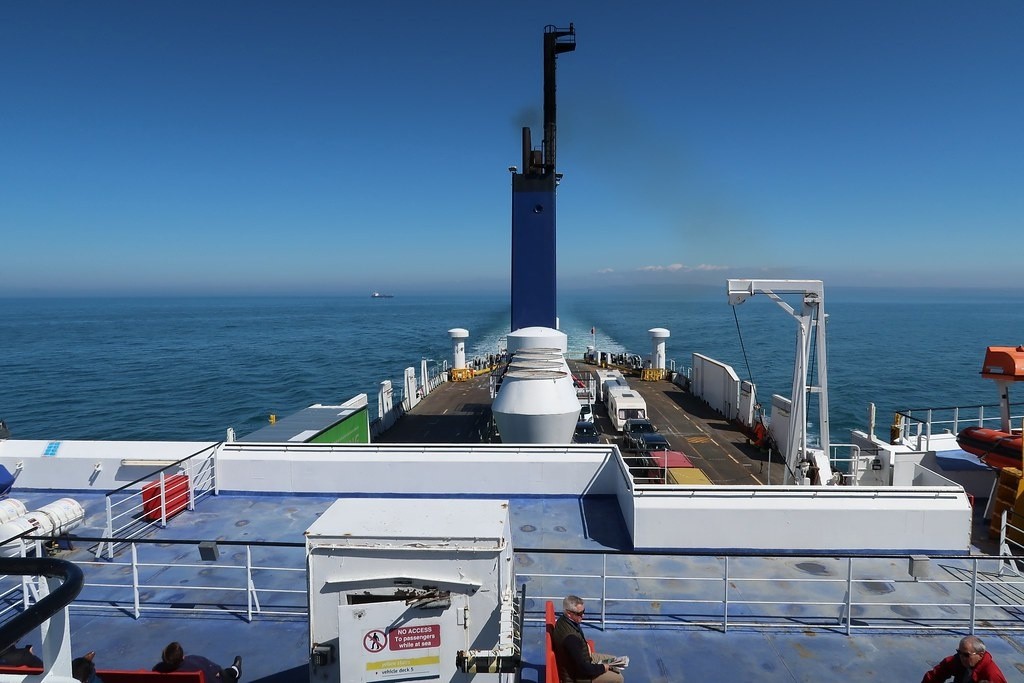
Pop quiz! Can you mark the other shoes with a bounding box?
[84,651,96,661]
[232,655,242,679]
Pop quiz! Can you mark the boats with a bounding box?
[371,292,394,298]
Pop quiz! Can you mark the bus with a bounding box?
[607,389,648,433]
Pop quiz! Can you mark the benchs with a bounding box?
[546,600,595,683]
[0,664,204,683]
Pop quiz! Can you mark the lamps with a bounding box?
[872,458,882,470]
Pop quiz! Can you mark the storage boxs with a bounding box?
[142,474,190,523]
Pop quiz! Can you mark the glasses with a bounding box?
[956,647,979,658]
[569,609,586,616]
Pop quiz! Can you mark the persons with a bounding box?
[0,648,103,683]
[153,642,242,683]
[552,594,624,683]
[920,635,1007,683]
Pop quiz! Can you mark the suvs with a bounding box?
[622,417,660,450]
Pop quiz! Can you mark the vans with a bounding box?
[663,468,716,485]
[635,432,672,474]
[594,368,631,409]
[646,450,694,485]
